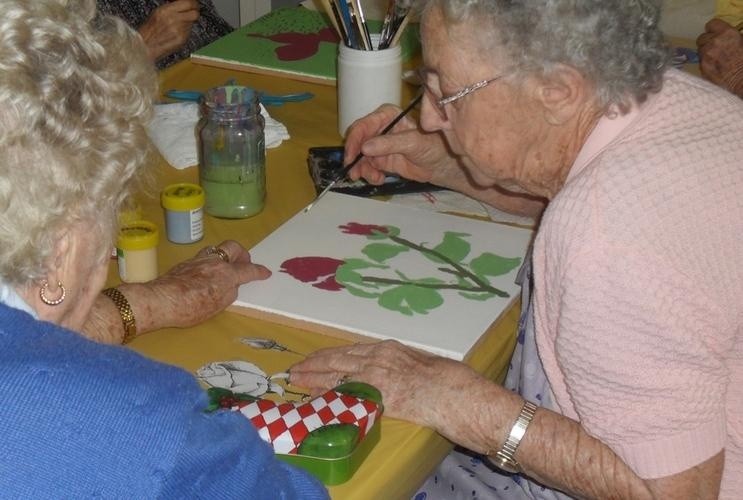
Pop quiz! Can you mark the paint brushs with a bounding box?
[304,91,423,214]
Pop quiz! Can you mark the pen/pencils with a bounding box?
[321,0,416,49]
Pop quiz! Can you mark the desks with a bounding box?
[89,36,702,500]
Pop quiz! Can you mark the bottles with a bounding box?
[194,86,267,218]
[160,182,205,245]
[110,219,160,284]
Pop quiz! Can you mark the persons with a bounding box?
[97,0,235,71]
[287,0,743,500]
[0,0,330,499]
[696,16,743,101]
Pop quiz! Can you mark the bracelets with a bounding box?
[102,287,138,345]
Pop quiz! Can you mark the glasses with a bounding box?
[411,60,524,122]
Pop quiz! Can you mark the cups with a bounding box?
[337,33,402,141]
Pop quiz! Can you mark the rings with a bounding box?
[338,374,351,386]
[213,246,230,265]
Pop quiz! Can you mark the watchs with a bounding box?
[487,401,537,474]
